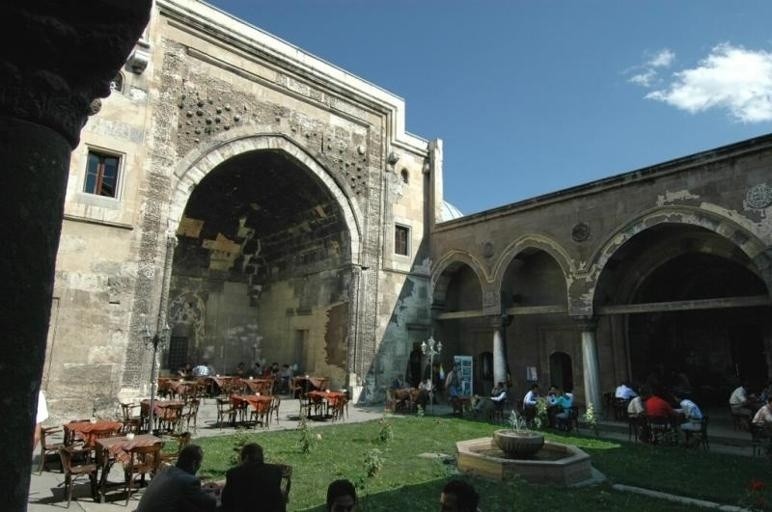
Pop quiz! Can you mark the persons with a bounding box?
[130,442,218,511]
[445,365,506,413]
[204,442,288,511]
[33,388,49,449]
[522,383,576,431]
[393,363,441,414]
[326,478,359,511]
[728,381,772,439]
[174,361,305,398]
[437,478,481,510]
[614,380,703,446]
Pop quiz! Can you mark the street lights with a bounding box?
[421,335,445,414]
[140,319,173,437]
